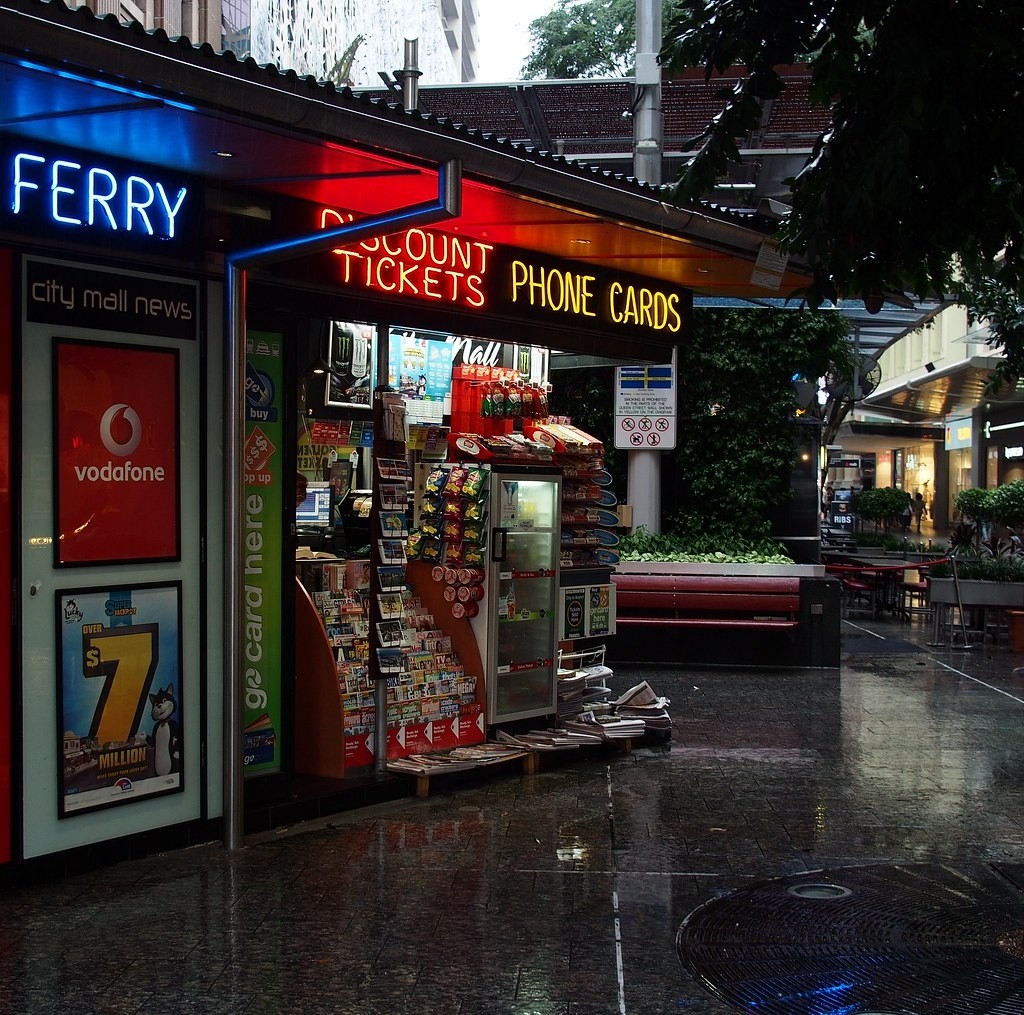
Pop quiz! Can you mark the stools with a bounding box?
[830,562,933,624]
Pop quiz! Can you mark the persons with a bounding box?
[296,470,308,508]
[842,484,928,531]
[959,503,997,540]
[823,487,834,521]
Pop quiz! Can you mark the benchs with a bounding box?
[605,573,802,666]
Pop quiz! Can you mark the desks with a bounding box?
[851,557,917,622]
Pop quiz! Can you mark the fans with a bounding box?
[825,352,882,403]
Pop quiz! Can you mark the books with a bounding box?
[312,559,480,736]
